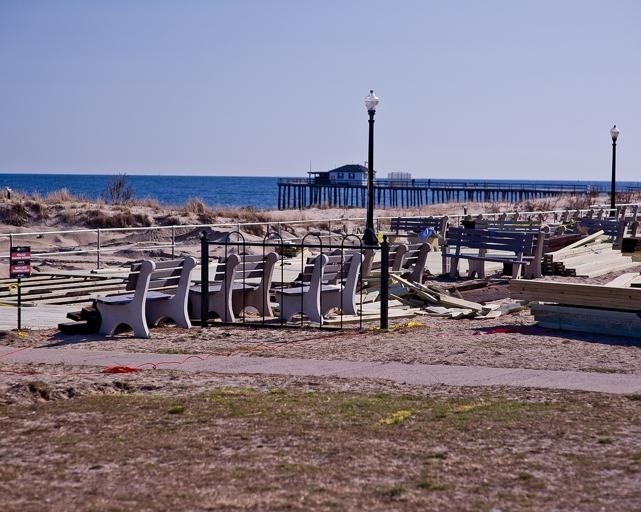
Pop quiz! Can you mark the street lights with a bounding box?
[363,91,380,245]
[609,124,620,208]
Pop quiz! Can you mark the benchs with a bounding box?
[383,217,445,253]
[277,252,365,325]
[441,229,530,281]
[462,228,544,279]
[96,256,197,339]
[190,252,279,323]
[393,243,431,283]
[574,216,626,248]
